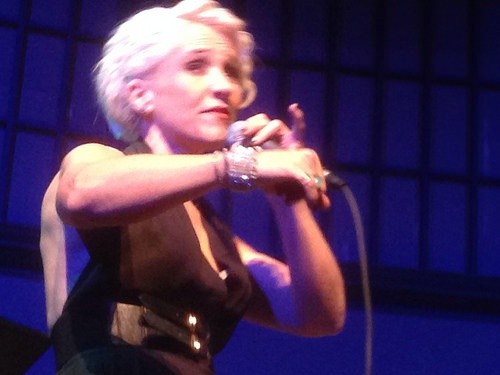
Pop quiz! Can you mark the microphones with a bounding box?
[227,119,342,190]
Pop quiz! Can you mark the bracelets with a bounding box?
[210,141,260,192]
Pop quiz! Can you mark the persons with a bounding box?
[39,0,346,375]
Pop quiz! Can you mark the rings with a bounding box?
[312,174,325,189]
